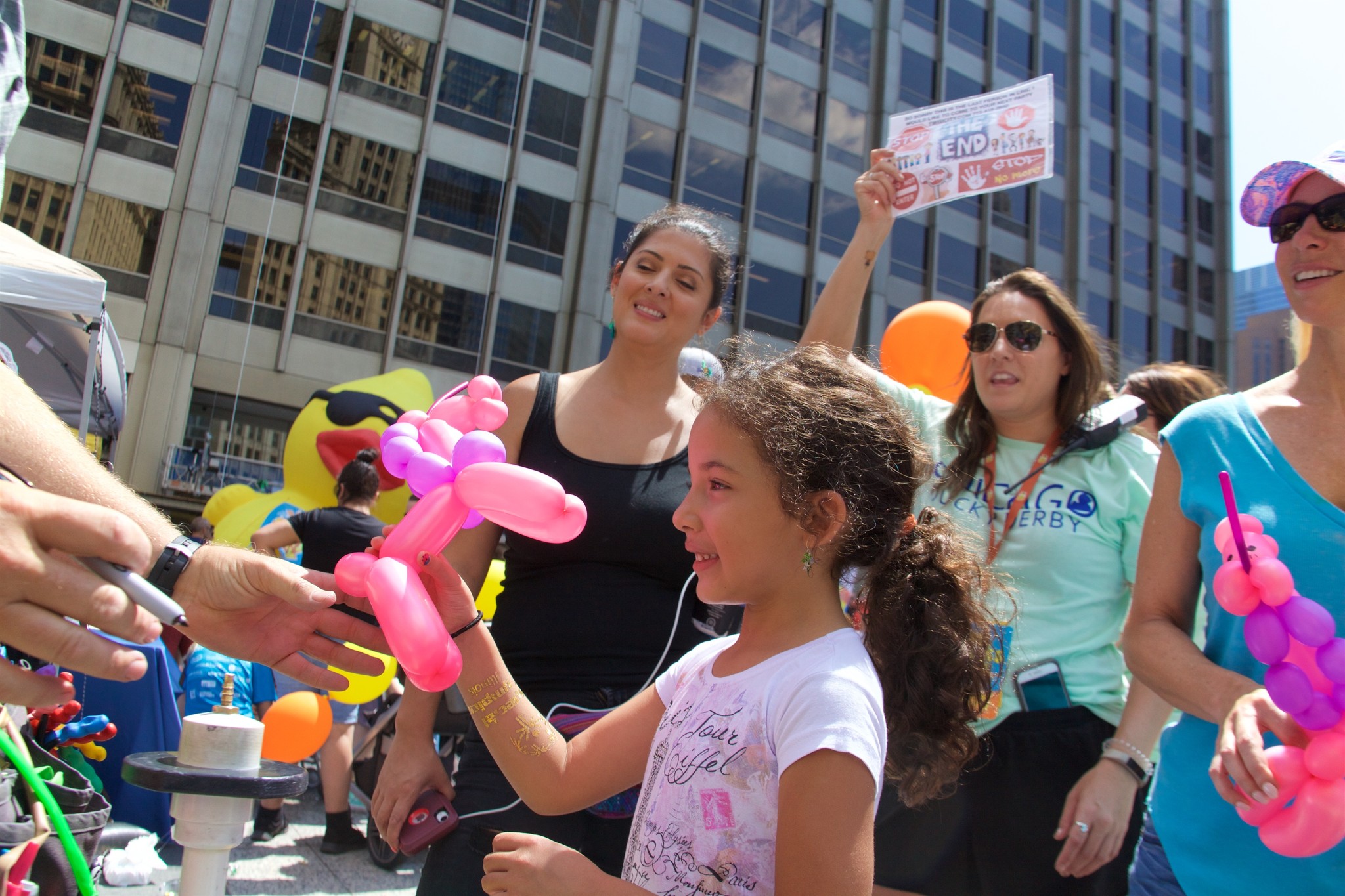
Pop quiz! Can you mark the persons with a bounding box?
[672,146,1345,896]
[183,642,278,722]
[369,207,745,895]
[364,332,1018,895]
[250,447,389,855]
[0,365,393,705]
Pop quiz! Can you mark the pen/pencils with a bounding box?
[0,462,189,629]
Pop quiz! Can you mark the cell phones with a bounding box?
[400,787,460,860]
[1013,658,1072,711]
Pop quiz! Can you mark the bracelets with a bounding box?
[450,610,484,639]
[147,534,202,598]
[1100,749,1147,788]
[1102,738,1154,776]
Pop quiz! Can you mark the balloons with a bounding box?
[327,640,397,705]
[1,664,118,896]
[1213,469,1343,858]
[260,690,333,764]
[880,300,975,407]
[333,371,587,691]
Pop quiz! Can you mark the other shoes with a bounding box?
[252,807,288,841]
[321,821,367,853]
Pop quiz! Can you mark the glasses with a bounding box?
[962,320,1061,353]
[1270,190,1345,245]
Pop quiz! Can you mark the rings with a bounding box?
[1075,821,1090,832]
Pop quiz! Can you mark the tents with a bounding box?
[0,220,127,444]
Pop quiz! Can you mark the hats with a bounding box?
[678,346,724,385]
[1240,149,1345,227]
